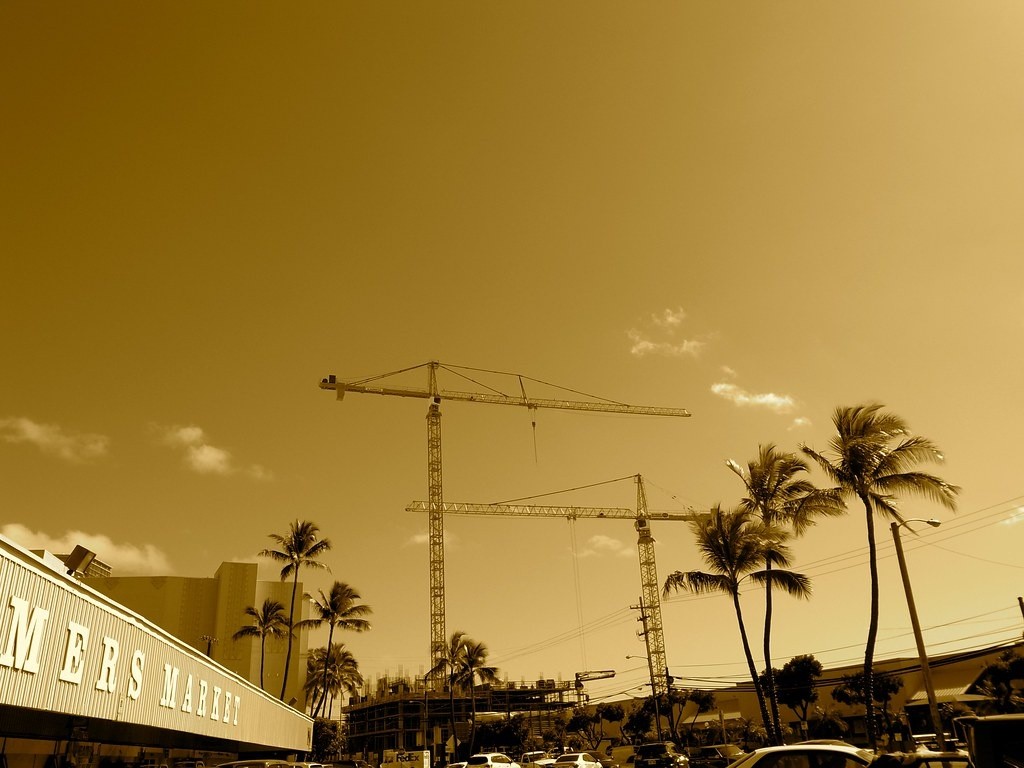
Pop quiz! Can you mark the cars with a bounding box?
[467,753,520,768]
[447,762,467,768]
[554,753,603,768]
[689,744,747,768]
[726,744,874,768]
[548,750,561,759]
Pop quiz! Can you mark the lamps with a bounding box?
[64,545,96,578]
[289,697,298,706]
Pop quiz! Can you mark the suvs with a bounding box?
[634,740,690,768]
[521,751,557,768]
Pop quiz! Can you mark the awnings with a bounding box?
[911,684,971,699]
[681,711,741,724]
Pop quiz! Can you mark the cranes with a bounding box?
[320,362,694,693]
[406,472,712,706]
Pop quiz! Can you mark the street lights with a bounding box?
[890,519,952,767]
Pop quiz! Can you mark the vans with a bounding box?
[215,760,375,768]
[567,751,620,768]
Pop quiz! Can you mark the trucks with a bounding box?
[379,751,431,768]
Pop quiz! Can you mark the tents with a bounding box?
[901,694,1001,719]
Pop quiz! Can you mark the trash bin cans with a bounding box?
[954,713,1024,768]
